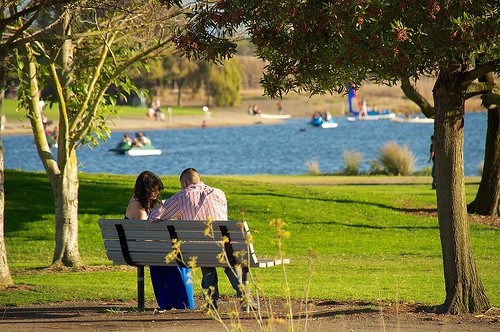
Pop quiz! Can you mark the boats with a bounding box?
[392,114,435,123]
[32,134,58,151]
[347,112,395,121]
[108,141,162,157]
[305,122,339,129]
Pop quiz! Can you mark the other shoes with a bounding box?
[241,301,261,311]
[206,301,218,310]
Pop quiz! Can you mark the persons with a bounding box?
[358,94,379,119]
[200,119,206,128]
[123,131,150,147]
[124,167,245,310]
[277,101,282,114]
[428,136,436,189]
[248,103,260,114]
[37,88,59,148]
[312,109,332,126]
[146,92,170,121]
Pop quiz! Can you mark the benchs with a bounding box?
[98,218,290,312]
